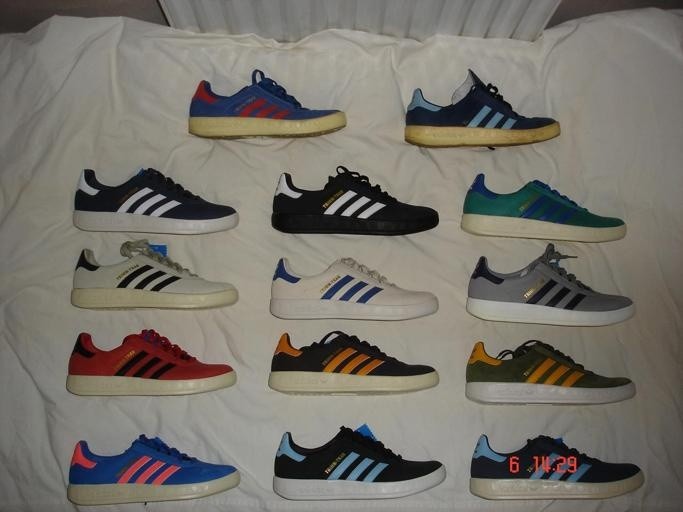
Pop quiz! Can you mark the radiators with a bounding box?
[158,0,564,43]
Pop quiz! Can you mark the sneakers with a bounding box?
[458,172,626,245]
[270,423,447,501]
[188,75,345,143]
[466,340,635,408]
[403,72,561,149]
[70,241,240,312]
[65,328,237,398]
[73,162,240,235]
[272,166,440,239]
[269,256,439,321]
[468,428,645,502]
[65,432,240,505]
[466,245,637,327]
[267,331,440,396]
